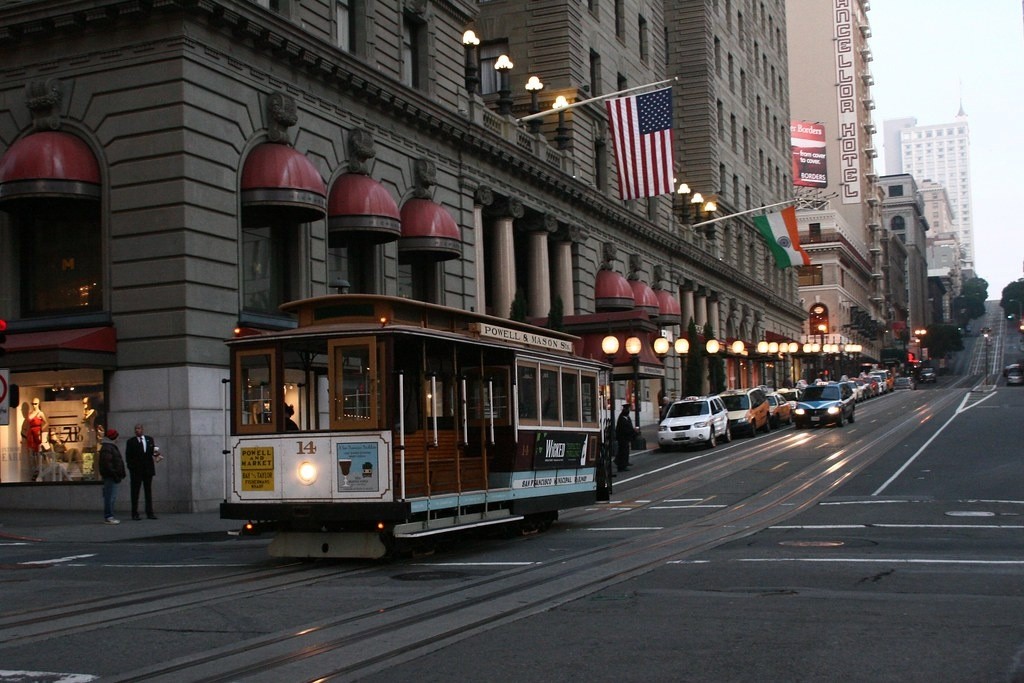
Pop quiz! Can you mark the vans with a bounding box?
[868,371,894,391]
[1007,368,1024,386]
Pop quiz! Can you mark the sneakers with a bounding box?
[104,516,121,525]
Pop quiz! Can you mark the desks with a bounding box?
[38,451,64,481]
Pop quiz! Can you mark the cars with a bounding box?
[658,396,731,451]
[766,389,803,430]
[795,381,857,429]
[846,376,885,403]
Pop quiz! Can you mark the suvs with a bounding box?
[894,367,937,391]
[718,389,770,439]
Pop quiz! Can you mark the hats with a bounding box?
[106,429,119,441]
[622,404,631,407]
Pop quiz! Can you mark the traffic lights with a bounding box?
[1008,315,1012,320]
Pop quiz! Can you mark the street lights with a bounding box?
[625,336,646,450]
[675,338,689,397]
[706,340,719,394]
[1009,299,1023,320]
[758,341,862,390]
[600,336,619,460]
[915,329,927,363]
[654,337,669,401]
[732,341,744,389]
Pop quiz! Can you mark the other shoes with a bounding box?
[612,458,619,466]
[618,467,630,471]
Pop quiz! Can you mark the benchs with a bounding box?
[392,430,494,494]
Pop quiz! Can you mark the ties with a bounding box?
[139,438,144,453]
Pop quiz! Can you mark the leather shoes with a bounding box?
[132,515,140,521]
[146,515,156,519]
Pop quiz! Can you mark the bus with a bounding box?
[220,279,615,560]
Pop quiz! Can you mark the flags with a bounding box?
[750,205,810,270]
[604,85,674,201]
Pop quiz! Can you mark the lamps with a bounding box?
[704,202,717,241]
[673,178,677,214]
[677,183,692,224]
[525,76,545,135]
[52,384,58,391]
[68,380,76,391]
[463,30,482,95]
[59,383,65,391]
[690,192,704,233]
[551,96,569,151]
[494,55,515,115]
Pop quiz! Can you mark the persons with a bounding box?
[125,423,160,521]
[783,375,792,388]
[660,397,672,423]
[24,397,49,482]
[285,403,299,431]
[49,431,83,481]
[98,429,126,525]
[613,404,639,467]
